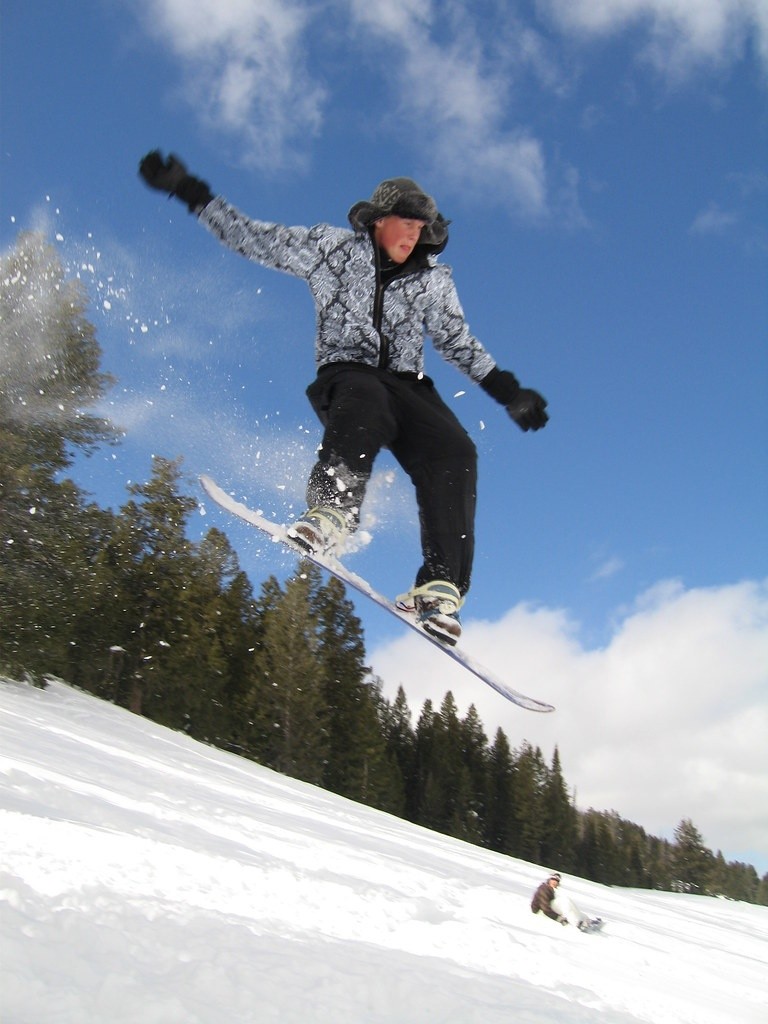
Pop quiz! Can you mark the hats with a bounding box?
[347,178,451,244]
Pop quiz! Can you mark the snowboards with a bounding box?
[193,471,562,720]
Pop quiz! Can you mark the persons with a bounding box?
[532,870,604,933]
[139,149,550,650]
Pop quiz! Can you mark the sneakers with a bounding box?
[413,579,462,645]
[288,505,348,554]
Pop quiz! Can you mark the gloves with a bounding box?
[480,370,550,433]
[139,149,210,215]
